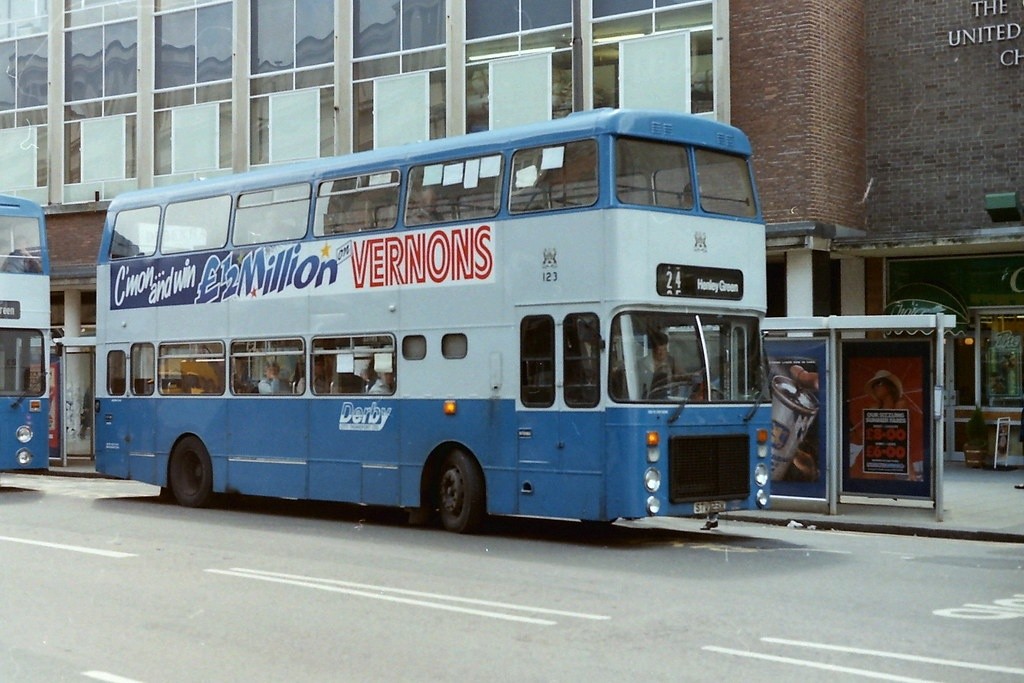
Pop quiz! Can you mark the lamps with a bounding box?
[984,192,1023,224]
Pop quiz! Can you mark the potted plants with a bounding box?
[963,408,989,470]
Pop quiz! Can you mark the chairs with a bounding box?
[110,373,361,395]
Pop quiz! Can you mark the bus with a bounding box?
[0,194,50,474]
[95,107,771,537]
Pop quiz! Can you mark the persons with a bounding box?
[635,330,687,399]
[290,357,394,394]
[3,235,43,274]
[256,363,291,394]
[233,359,255,393]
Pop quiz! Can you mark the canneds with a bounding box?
[769,375,819,481]
